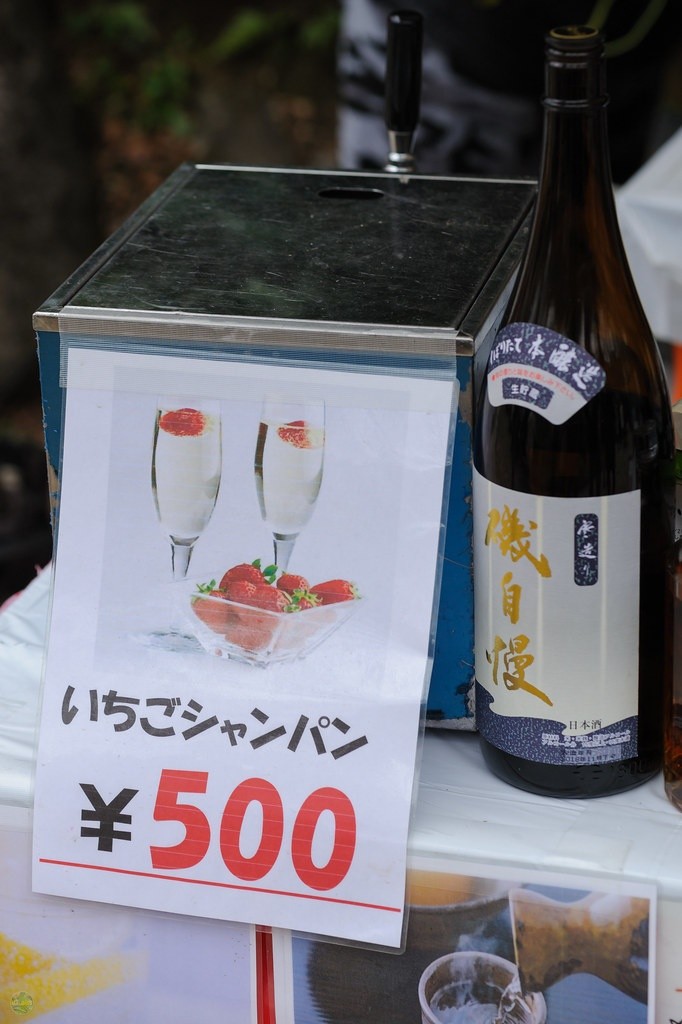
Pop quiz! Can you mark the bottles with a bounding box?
[472,26,676,800]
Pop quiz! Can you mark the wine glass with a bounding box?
[129,394,223,663]
[255,399,326,586]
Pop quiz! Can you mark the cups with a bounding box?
[417,950,547,1024]
[663,537,682,815]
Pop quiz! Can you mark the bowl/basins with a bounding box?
[170,562,367,668]
[308,870,522,1024]
